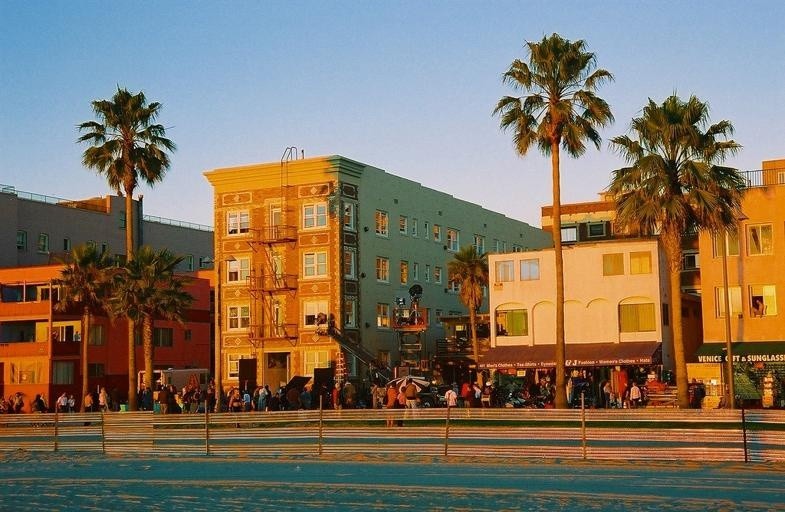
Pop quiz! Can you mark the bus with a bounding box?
[137,367,209,404]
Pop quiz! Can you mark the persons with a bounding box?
[52,330,60,341]
[752,299,765,318]
